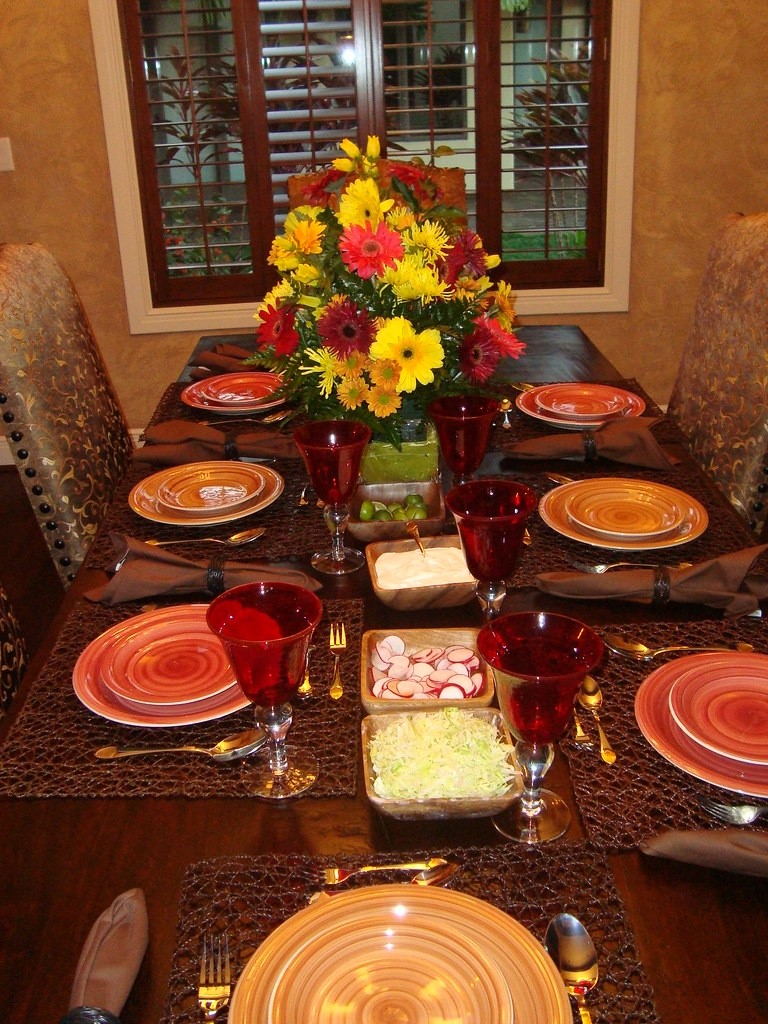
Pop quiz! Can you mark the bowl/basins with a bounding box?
[347,476,446,542]
[366,534,478,611]
[668,658,768,766]
[360,626,495,714]
[201,375,282,406]
[534,386,634,421]
[565,484,686,543]
[155,466,266,517]
[361,707,524,822]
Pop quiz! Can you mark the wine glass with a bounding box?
[293,419,372,575]
[445,479,538,630]
[205,579,324,799]
[477,611,604,843]
[428,396,500,488]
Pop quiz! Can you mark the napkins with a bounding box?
[79,530,325,608]
[186,341,266,381]
[638,827,768,879]
[129,418,304,466]
[502,415,683,473]
[68,886,149,1018]
[535,543,768,624]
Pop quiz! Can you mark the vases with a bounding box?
[359,418,439,485]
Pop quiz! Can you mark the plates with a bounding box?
[633,651,768,799]
[128,460,285,528]
[72,604,282,728]
[538,478,709,551]
[227,884,574,1023]
[180,371,292,416]
[515,382,647,431]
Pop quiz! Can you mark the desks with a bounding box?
[0,323,768,1024]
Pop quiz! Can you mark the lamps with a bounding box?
[513,5,530,34]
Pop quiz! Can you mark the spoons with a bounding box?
[94,729,269,763]
[600,632,755,661]
[144,528,266,548]
[543,913,599,1024]
[578,676,616,765]
[292,483,310,507]
[294,644,320,704]
[543,471,574,485]
[198,410,292,425]
[499,398,514,430]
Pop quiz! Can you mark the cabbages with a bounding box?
[366,706,523,800]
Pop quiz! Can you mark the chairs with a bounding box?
[664,209,768,547]
[1,240,136,589]
[284,156,468,233]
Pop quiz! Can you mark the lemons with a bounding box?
[359,495,431,521]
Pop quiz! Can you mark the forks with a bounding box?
[329,622,347,700]
[198,934,230,1024]
[564,555,680,574]
[573,708,597,752]
[699,797,768,825]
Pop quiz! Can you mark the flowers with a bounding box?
[240,135,527,453]
